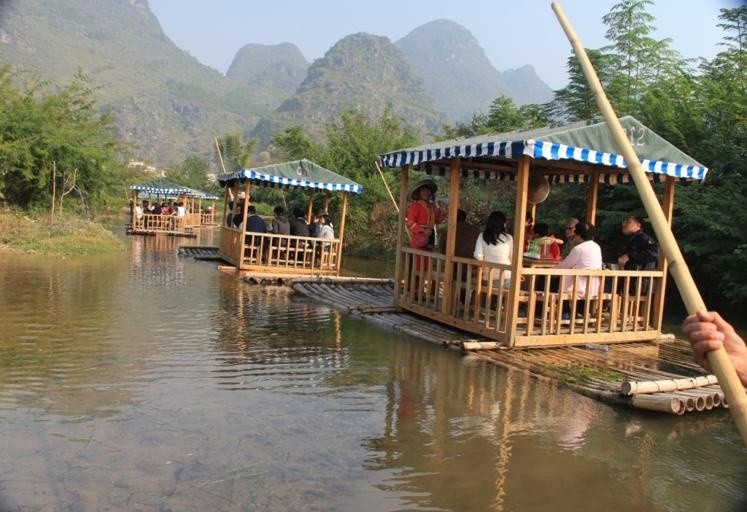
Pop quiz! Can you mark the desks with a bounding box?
[521,255,606,309]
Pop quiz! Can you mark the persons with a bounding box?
[131,199,213,231]
[682,309,747,391]
[223,189,336,266]
[404,176,660,326]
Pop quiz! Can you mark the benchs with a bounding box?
[136,219,184,230]
[534,290,649,327]
[415,268,534,329]
[244,240,336,267]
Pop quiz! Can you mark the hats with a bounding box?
[526,172,551,206]
[411,178,437,201]
[237,191,251,200]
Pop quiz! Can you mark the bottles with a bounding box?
[527,236,552,258]
